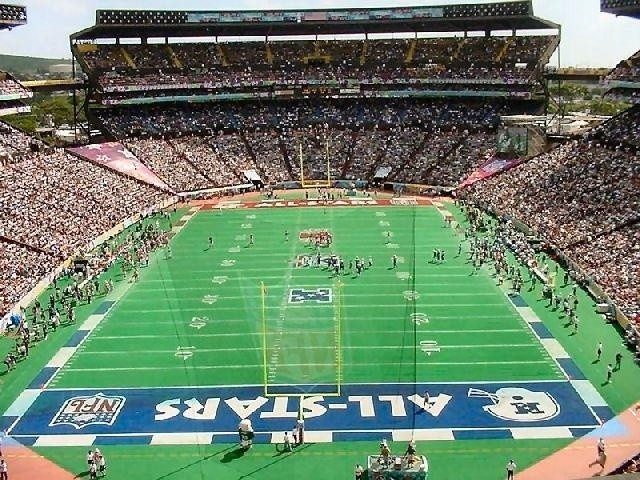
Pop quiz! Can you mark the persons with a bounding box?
[283,432,292,452]
[292,425,299,444]
[237,415,254,453]
[311,241,373,278]
[387,231,391,243]
[431,249,446,265]
[0,459,8,480]
[76,38,558,206]
[589,402,640,480]
[451,104,640,387]
[0,72,30,101]
[352,441,417,480]
[608,51,640,84]
[87,447,107,480]
[392,255,398,267]
[284,230,289,242]
[1,122,182,373]
[208,236,213,248]
[506,460,516,480]
[423,390,431,409]
[247,234,254,247]
